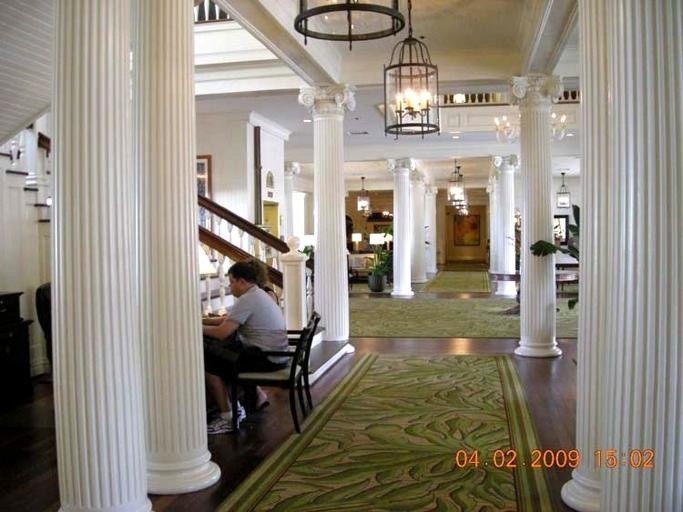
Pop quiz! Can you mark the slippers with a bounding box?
[249,402,269,413]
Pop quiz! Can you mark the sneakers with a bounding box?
[207,405,246,435]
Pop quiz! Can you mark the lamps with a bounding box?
[382,1,442,139]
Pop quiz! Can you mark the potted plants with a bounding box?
[367,239,395,292]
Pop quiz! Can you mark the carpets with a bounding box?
[348,270,578,339]
[215,351,559,512]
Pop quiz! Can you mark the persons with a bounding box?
[202,262,290,435]
[244,258,281,411]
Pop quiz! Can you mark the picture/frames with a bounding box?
[554,214,569,245]
[196,154,213,263]
[453,215,480,246]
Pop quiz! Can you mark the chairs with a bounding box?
[228,309,321,435]
